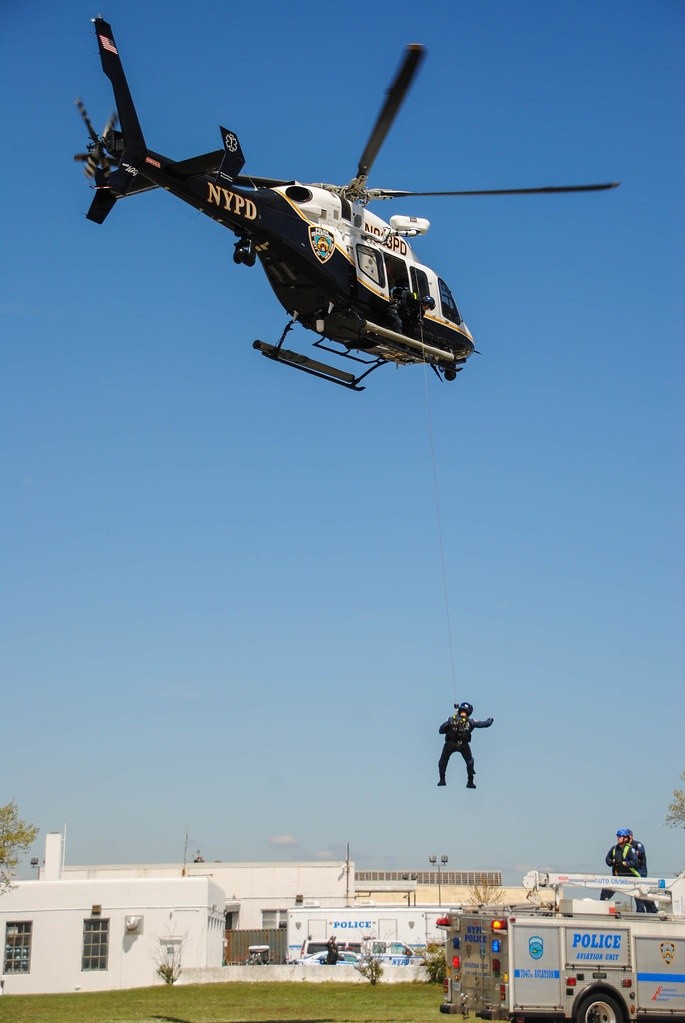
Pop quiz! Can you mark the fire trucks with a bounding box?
[433,870,684,1023]
[285,904,465,964]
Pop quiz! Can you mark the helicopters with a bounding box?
[70,12,627,392]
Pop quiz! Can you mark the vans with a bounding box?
[300,936,425,967]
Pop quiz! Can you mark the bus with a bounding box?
[248,945,272,965]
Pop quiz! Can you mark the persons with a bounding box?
[388,286,435,336]
[600,828,658,913]
[437,702,494,789]
[326,936,344,965]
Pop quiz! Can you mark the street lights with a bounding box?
[429,855,449,908]
[402,874,419,906]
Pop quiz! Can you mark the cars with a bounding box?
[292,950,367,965]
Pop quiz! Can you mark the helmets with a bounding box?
[625,828,632,835]
[458,703,473,717]
[616,828,629,838]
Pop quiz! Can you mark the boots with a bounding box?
[466,776,476,788]
[437,775,446,786]
